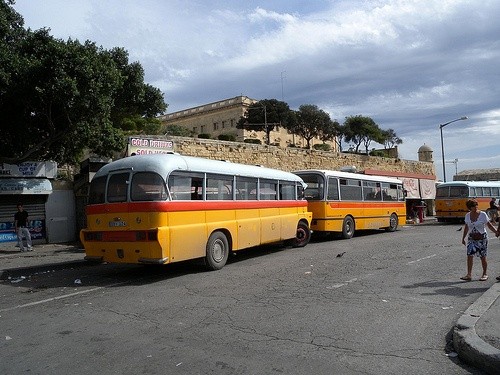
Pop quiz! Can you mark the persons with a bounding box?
[460,198,500,281]
[14,205,33,253]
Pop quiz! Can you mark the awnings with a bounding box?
[0,177,54,195]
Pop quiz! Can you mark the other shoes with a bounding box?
[479,276,489,281]
[459,276,472,281]
[492,221,497,226]
[496,276,500,281]
[20,248,25,253]
[27,248,34,251]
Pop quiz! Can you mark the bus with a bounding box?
[292,165,408,239]
[79,152,312,272]
[434,180,500,222]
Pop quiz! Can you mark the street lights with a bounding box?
[439,116,468,181]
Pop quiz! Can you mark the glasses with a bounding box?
[471,203,479,207]
